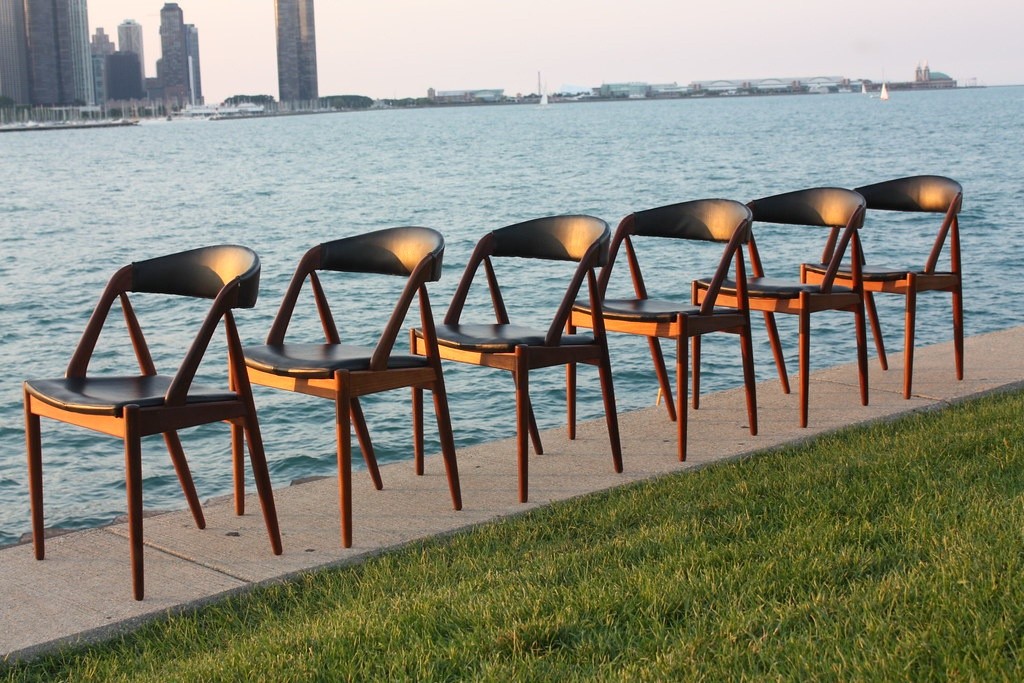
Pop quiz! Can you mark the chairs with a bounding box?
[411,213,624,502]
[798,172,965,400]
[227,226,463,549]
[23,243,281,603]
[691,187,869,428]
[565,197,758,463]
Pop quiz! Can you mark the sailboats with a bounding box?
[880,82,888,100]
[861,82,867,95]
[538,88,549,106]
